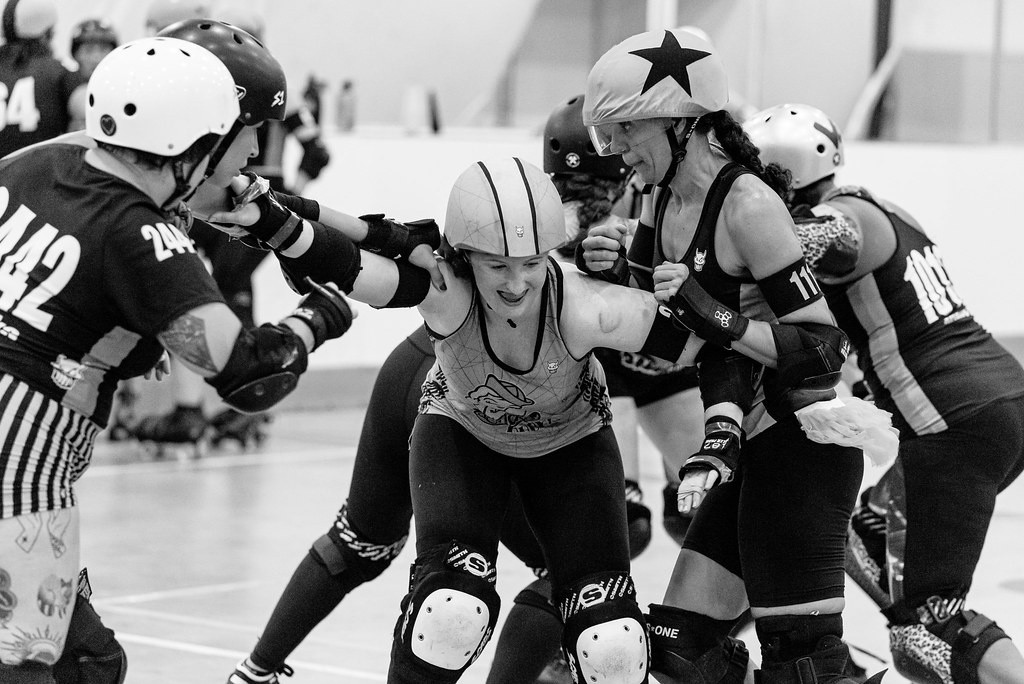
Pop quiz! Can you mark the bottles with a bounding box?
[338,81,355,130]
[304,75,320,124]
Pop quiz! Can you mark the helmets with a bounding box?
[741,103,844,189]
[71,19,120,57]
[582,29,729,126]
[153,18,287,126]
[444,157,566,258]
[2,0,59,43]
[544,94,633,185]
[85,37,241,156]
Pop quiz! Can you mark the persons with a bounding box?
[1,0,1024,683]
